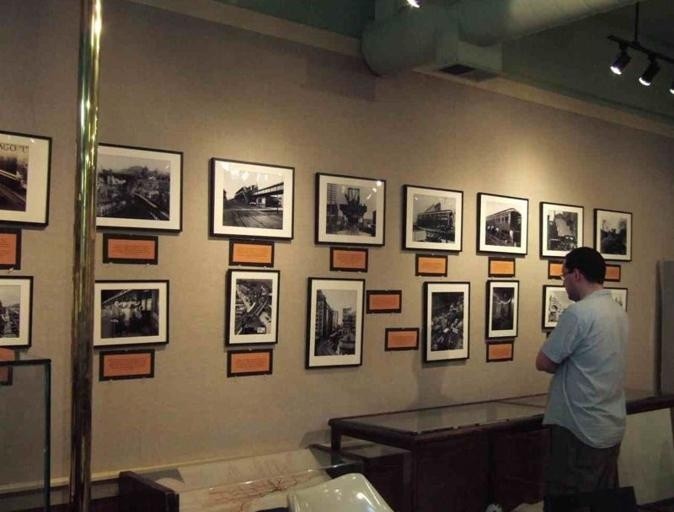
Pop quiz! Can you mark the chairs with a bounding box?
[119,472,174,512]
[405,427,551,512]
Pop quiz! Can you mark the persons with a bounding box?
[536,247,630,495]
[103,302,142,336]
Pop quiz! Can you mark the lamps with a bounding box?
[606,0,674,94]
